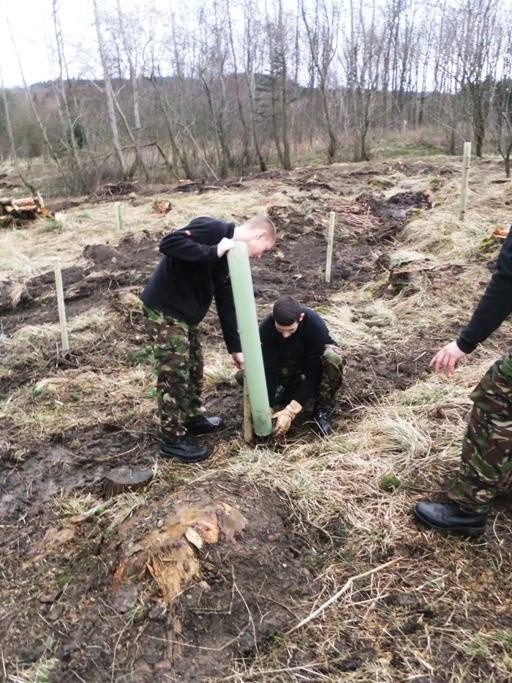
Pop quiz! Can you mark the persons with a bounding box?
[234,292,347,440]
[409,222,512,538]
[137,213,278,462]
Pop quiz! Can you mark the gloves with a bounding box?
[272,399,303,437]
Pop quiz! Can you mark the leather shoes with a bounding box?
[413,501,485,538]
[312,407,331,434]
[184,415,224,433]
[158,434,211,462]
